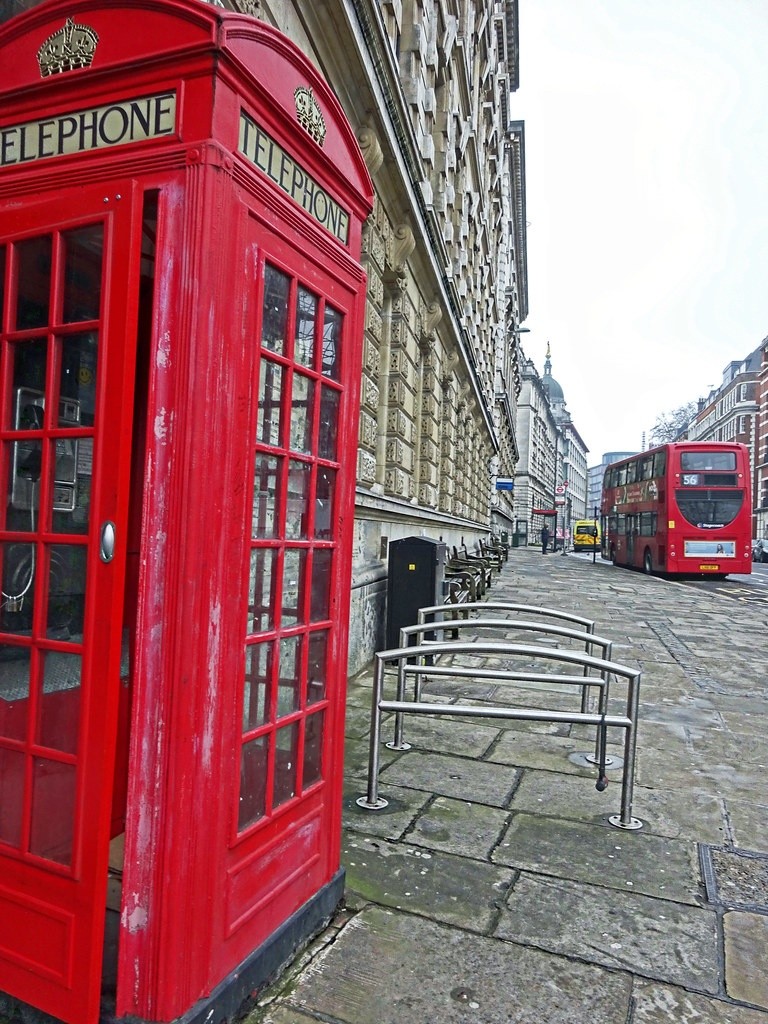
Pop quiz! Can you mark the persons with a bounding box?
[542,523,550,555]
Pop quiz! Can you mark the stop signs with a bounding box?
[557,486,564,493]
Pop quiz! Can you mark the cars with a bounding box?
[750,538,768,563]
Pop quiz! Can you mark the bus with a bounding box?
[600,440,752,580]
[573,520,601,553]
